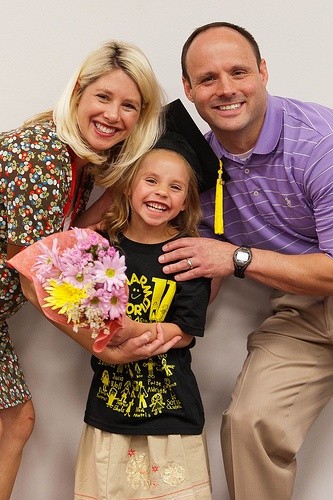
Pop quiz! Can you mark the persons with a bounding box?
[0,40,182,500]
[74,98,211,500]
[158,22,333,500]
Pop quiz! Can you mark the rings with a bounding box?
[187,257,192,269]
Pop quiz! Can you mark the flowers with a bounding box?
[30,227,127,339]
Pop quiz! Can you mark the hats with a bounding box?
[119,98,232,234]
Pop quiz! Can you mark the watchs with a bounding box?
[233,244,252,279]
